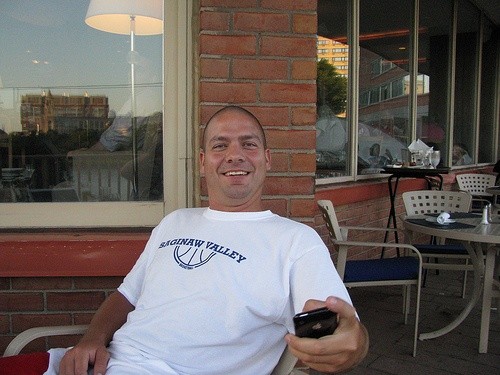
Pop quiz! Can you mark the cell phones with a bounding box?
[294,306,338,339]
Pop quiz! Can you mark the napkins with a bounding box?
[437,212,455,224]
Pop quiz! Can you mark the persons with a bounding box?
[57,105,368,375]
[65,66,160,202]
[368,143,396,166]
[453,144,472,165]
[315,97,347,151]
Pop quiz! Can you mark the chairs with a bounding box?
[66,110,163,201]
[317,174,496,358]
[0,90,34,202]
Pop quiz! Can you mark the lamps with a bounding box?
[84,0,163,202]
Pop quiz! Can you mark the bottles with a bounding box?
[410,150,421,166]
[481,203,492,224]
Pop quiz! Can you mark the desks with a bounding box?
[380,166,450,288]
[404,212,500,354]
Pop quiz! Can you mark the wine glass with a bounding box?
[422,150,440,169]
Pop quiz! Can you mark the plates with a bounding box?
[425,218,456,225]
